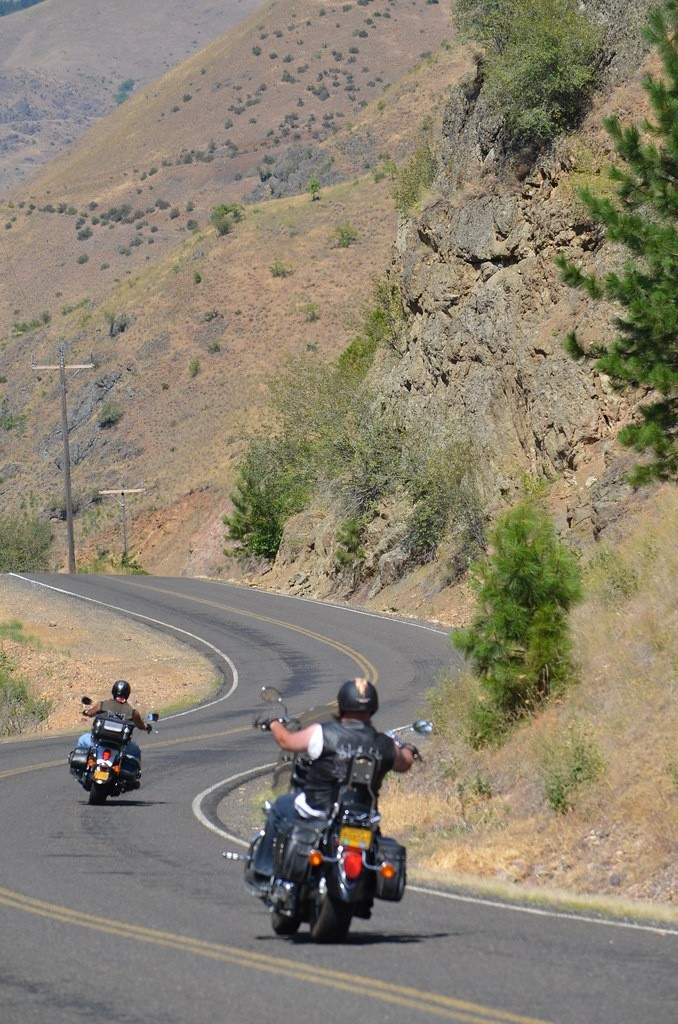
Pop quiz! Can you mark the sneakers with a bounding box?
[243,869,272,896]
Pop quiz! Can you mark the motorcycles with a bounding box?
[222,686,433,942]
[68,695,159,806]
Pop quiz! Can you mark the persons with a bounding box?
[68,681,154,786]
[244,678,416,897]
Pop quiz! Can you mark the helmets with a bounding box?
[335,678,380,715]
[111,680,131,702]
